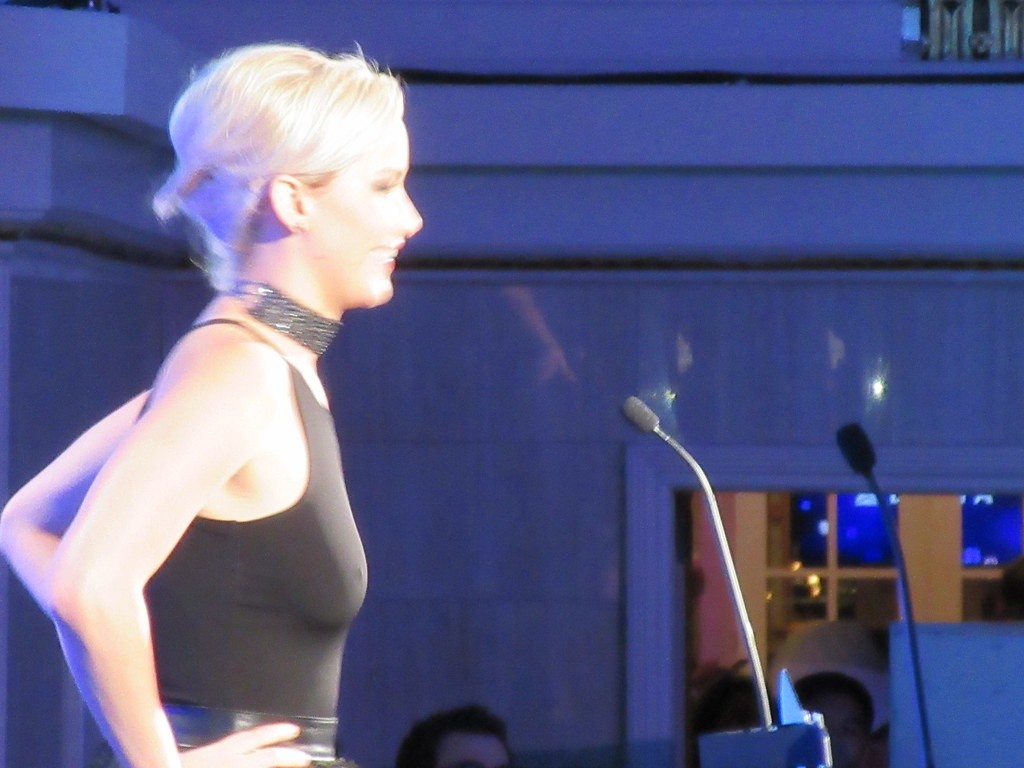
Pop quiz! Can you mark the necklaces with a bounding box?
[229,276,341,353]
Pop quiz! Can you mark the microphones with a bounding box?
[622,395,776,726]
[833,420,937,767]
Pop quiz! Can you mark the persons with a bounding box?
[688,670,889,767]
[0,45,424,768]
[396,705,511,768]
